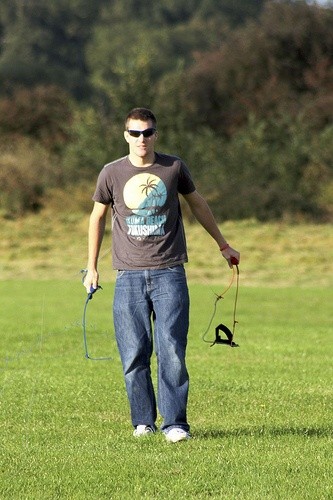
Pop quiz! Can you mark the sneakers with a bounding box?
[134,424,154,438]
[167,428,191,443]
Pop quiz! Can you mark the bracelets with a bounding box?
[219,243,230,251]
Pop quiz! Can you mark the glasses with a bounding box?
[127,128,156,138]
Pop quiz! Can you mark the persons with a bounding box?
[84,107,241,442]
